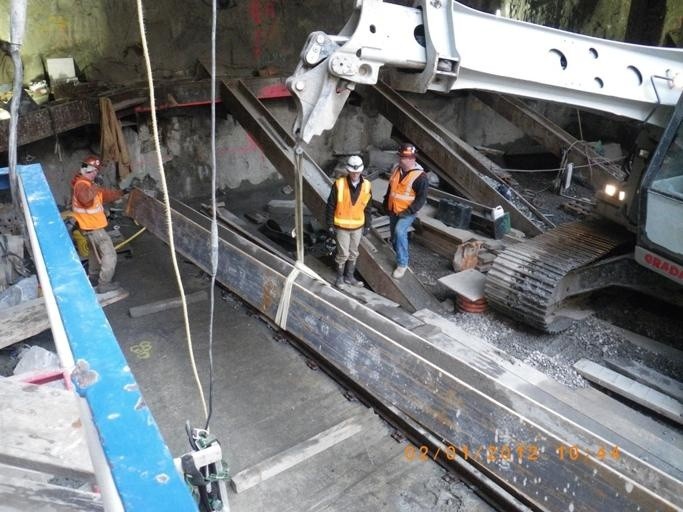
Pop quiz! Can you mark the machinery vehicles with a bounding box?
[288,1,682,338]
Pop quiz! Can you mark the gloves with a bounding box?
[329,228,336,233]
[123,186,136,195]
[94,175,103,185]
[364,228,370,235]
[398,210,408,219]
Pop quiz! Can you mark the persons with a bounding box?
[380,142,428,280]
[324,156,373,289]
[71,156,137,295]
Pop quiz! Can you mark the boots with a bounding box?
[336,263,345,288]
[346,261,364,287]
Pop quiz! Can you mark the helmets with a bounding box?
[82,157,102,171]
[398,144,415,158]
[346,156,364,172]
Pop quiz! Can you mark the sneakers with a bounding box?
[392,265,408,278]
[89,276,119,293]
